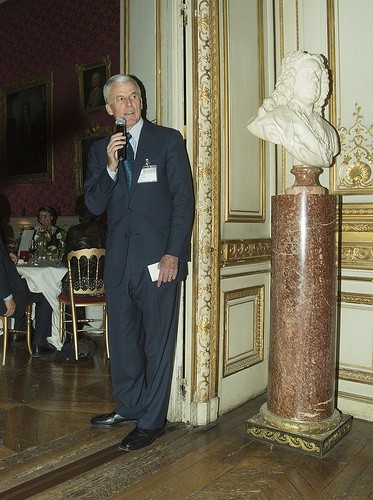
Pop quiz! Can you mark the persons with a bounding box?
[0,194,104,358]
[84,74,196,451]
[247,50,340,168]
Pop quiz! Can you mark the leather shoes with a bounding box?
[89,410,137,428]
[10,333,34,345]
[32,345,56,358]
[118,426,165,451]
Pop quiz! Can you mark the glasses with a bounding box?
[39,214,51,219]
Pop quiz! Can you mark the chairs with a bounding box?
[0,302,33,366]
[58,248,110,360]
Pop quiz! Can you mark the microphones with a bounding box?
[115,117,127,162]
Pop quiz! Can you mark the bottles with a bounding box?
[20,226,24,236]
[29,226,32,230]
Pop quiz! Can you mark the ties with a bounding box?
[122,133,134,195]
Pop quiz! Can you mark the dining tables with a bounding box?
[0,262,103,351]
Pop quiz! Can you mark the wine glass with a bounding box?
[29,239,36,263]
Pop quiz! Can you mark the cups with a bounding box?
[20,251,29,261]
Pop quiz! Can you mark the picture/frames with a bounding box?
[75,55,113,116]
[70,120,120,198]
[0,70,55,187]
